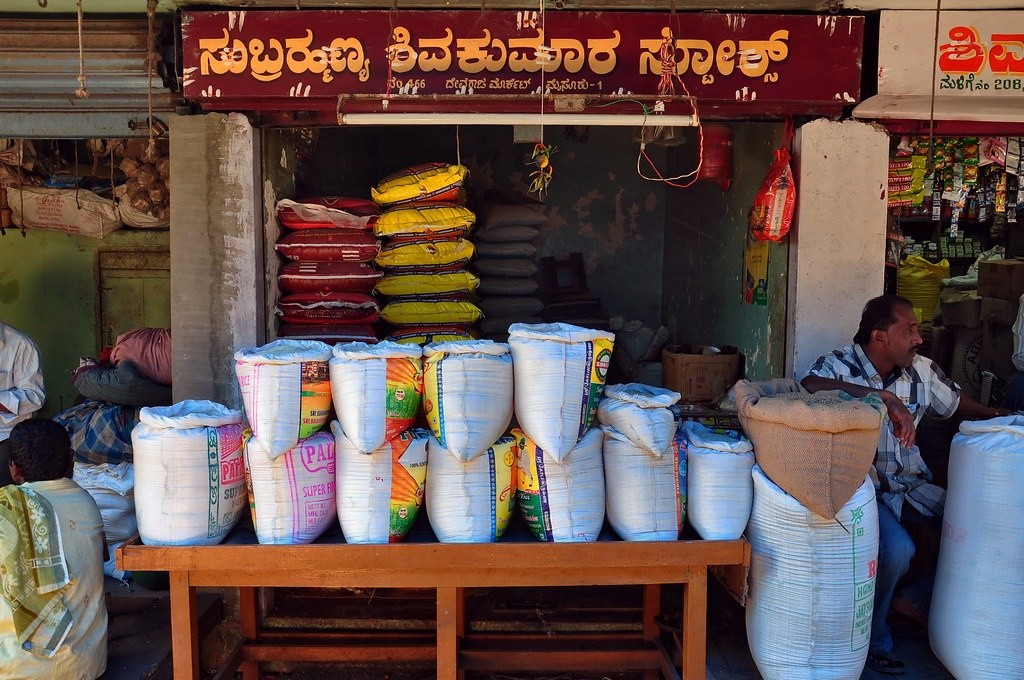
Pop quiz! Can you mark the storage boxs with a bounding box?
[977,259,1024,302]
[661,344,739,403]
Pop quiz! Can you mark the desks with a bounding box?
[116,531,753,680]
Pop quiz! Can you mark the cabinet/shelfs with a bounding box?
[884,215,1024,277]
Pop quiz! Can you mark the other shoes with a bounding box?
[868,649,906,673]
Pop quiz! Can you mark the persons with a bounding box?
[801,294,1013,674]
[0,319,47,489]
[0,416,109,680]
